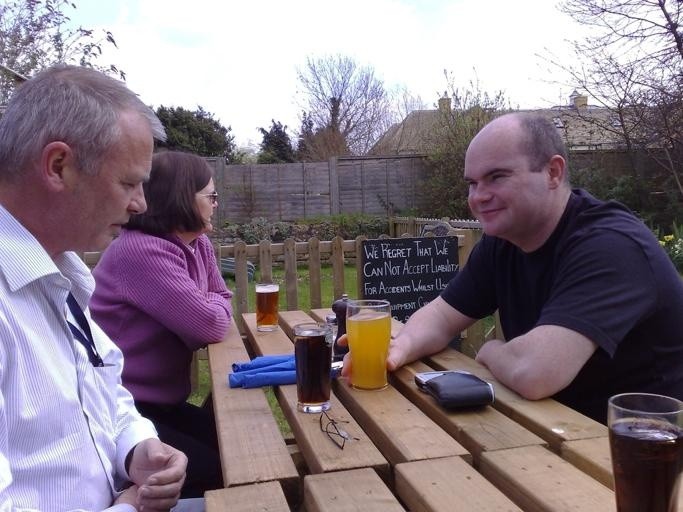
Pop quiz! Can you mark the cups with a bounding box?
[608,392,683,511]
[293,299,392,413]
[255,282,280,331]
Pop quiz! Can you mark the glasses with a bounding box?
[320,411,359,449]
[194,191,218,204]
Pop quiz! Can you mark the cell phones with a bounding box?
[414,370,475,392]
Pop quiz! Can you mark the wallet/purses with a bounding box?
[425,371,495,409]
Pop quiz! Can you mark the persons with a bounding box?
[85,148,235,500]
[1,62,191,512]
[332,111,681,444]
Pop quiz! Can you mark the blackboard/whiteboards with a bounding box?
[361,236,462,353]
